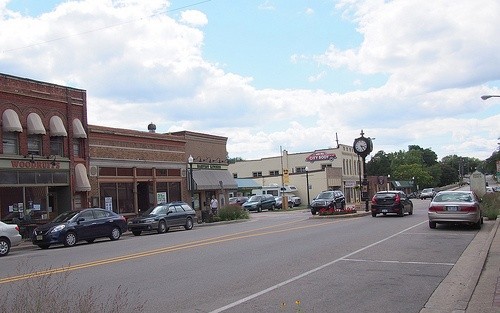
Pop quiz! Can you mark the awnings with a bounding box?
[235,179,262,190]
[188,170,238,191]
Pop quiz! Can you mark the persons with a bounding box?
[211,195,218,216]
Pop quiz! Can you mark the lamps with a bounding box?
[50,155,57,164]
[26,153,35,163]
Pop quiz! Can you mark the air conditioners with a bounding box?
[90,166,97,176]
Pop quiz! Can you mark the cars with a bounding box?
[486,181,500,192]
[127,200,197,236]
[31,207,128,249]
[229,192,302,210]
[0,220,23,257]
[420,188,437,200]
[427,190,484,230]
[242,194,276,213]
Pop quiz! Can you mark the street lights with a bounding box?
[412,176,415,191]
[188,154,194,210]
[305,166,311,209]
[387,174,391,190]
[24,152,35,163]
[47,154,56,165]
[481,95,500,100]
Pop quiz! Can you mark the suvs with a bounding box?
[370,190,414,217]
[310,190,345,215]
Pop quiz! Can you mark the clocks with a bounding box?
[354,139,368,152]
